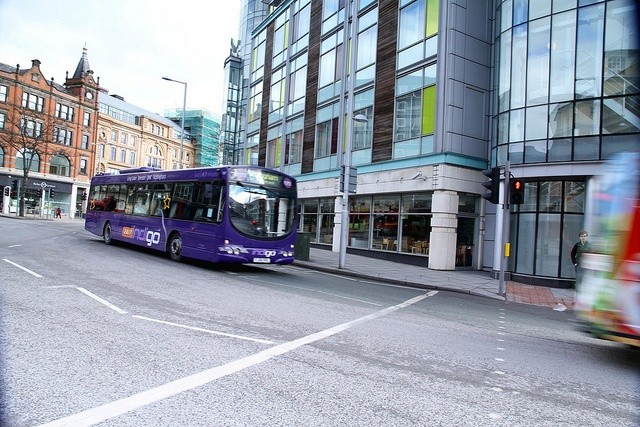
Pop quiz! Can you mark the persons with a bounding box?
[569,232,591,281]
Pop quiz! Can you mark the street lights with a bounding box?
[339,111,369,270]
[162,74,188,170]
[98,133,116,175]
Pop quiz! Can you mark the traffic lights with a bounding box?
[10,192,17,199]
[81,193,87,201]
[11,179,17,191]
[5,187,9,197]
[36,189,41,198]
[50,188,54,198]
[509,177,526,205]
[479,167,499,205]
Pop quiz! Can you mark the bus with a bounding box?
[84,167,298,269]
[570,152,637,347]
